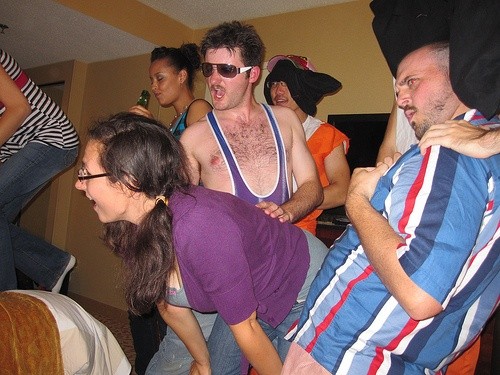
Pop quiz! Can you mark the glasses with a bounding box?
[77,167,109,184]
[266,80,287,89]
[200,62,253,79]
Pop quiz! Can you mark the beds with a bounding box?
[0,289,133,375]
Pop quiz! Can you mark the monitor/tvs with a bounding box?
[319,113,390,224]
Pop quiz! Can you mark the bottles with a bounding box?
[137,89,150,109]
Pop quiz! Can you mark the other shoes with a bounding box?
[50,253,76,293]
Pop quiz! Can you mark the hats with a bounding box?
[267,54,316,72]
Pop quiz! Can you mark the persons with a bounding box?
[73,111,332,375]
[368,0,500,170]
[0,45,81,294]
[126,42,214,375]
[177,20,325,224]
[263,54,351,237]
[277,38,500,375]
